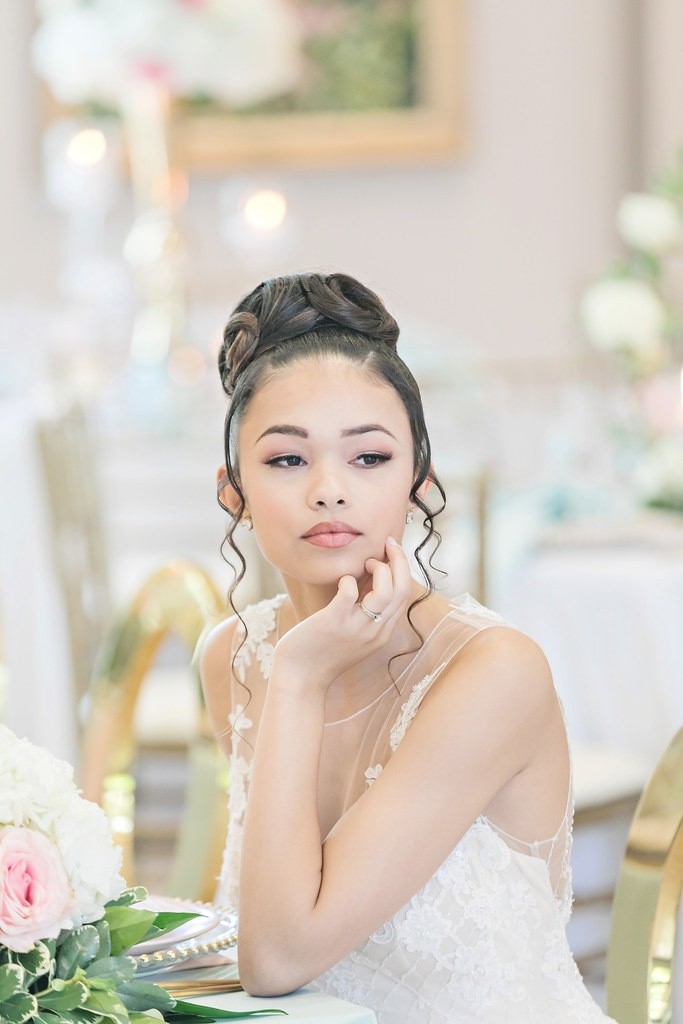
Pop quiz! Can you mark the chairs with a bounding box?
[84,565,233,904]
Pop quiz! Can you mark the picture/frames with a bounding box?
[38,0,457,168]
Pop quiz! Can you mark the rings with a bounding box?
[361,602,381,621]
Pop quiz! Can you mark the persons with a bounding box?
[194,273,622,1024]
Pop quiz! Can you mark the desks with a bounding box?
[144,989,381,1024]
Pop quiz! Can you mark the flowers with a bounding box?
[0,715,290,1024]
